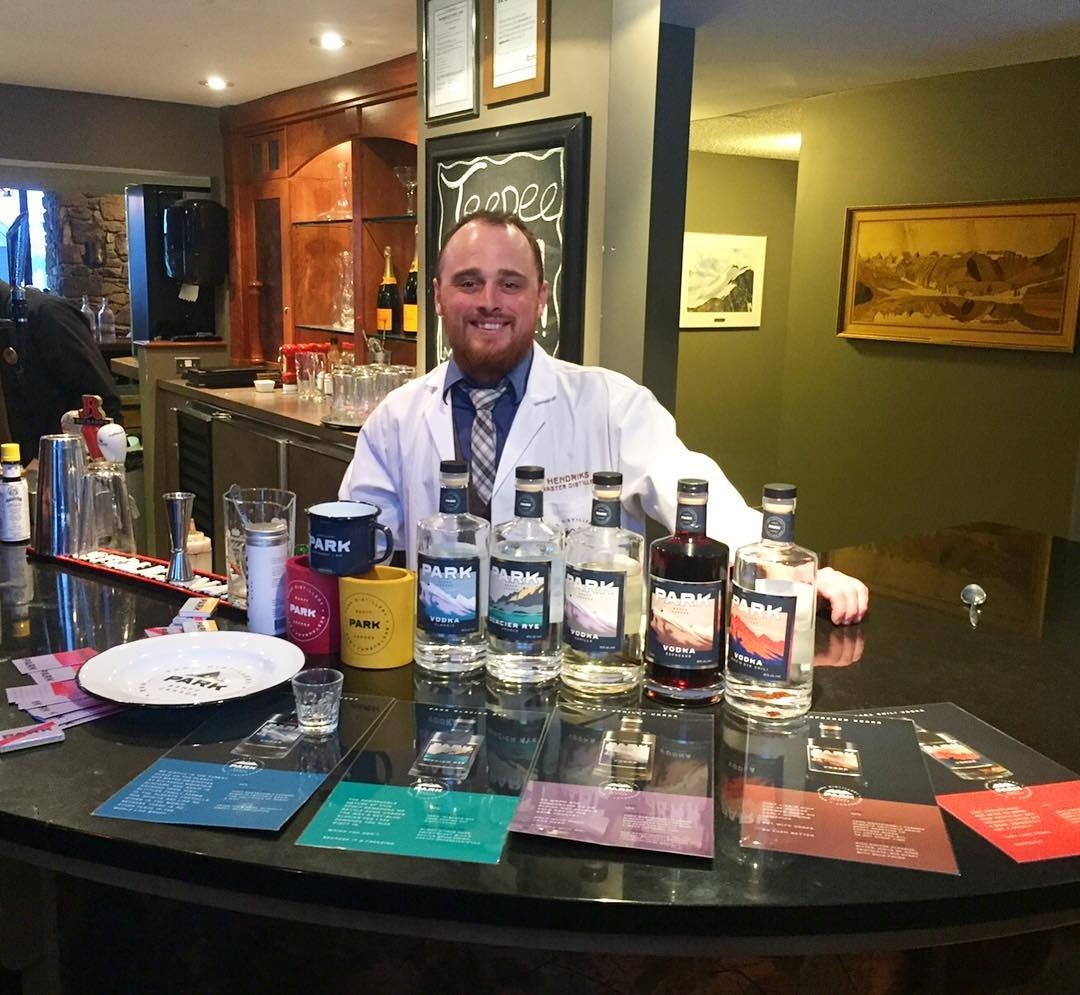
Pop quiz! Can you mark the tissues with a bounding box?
[163,198,228,303]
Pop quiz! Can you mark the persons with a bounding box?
[334,206,871,634]
[0,277,126,496]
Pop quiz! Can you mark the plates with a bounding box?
[75,629,305,709]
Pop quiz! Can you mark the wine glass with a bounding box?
[393,166,417,215]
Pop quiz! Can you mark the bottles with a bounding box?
[185,517,214,574]
[411,680,812,909]
[0,543,34,637]
[722,482,817,722]
[484,464,567,692]
[806,710,1014,782]
[243,521,291,636]
[0,443,31,542]
[80,294,116,340]
[332,224,418,339]
[413,459,490,679]
[561,471,646,702]
[640,478,731,708]
[229,707,304,760]
[316,160,354,221]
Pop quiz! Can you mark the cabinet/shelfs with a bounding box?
[220,51,419,371]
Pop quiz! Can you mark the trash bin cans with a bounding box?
[125,434,147,555]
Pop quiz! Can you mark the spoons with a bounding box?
[959,582,986,628]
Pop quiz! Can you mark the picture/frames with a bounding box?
[817,523,1055,646]
[836,198,1080,354]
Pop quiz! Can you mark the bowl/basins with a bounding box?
[253,380,276,392]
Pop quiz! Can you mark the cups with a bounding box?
[279,342,418,426]
[31,432,138,567]
[284,499,416,670]
[37,567,138,655]
[161,491,196,581]
[290,667,344,739]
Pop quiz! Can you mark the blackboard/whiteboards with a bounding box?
[425,112,586,374]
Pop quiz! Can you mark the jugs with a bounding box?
[221,485,296,610]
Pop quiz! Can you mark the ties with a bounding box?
[456,380,509,506]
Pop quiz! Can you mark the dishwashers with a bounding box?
[176,402,216,575]
